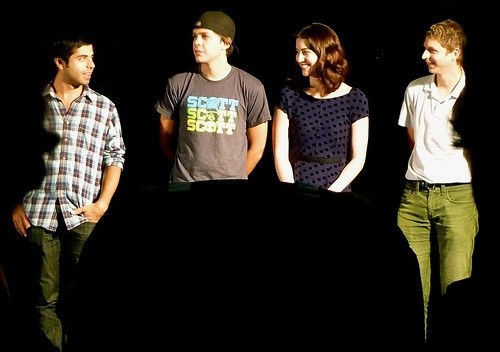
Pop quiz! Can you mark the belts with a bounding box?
[405,179,472,191]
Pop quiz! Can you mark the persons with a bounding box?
[424,21,499,351]
[0,51,60,348]
[397,19,479,342]
[155,11,272,187]
[273,22,369,193]
[10,35,127,345]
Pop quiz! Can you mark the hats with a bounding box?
[193,11,239,55]
